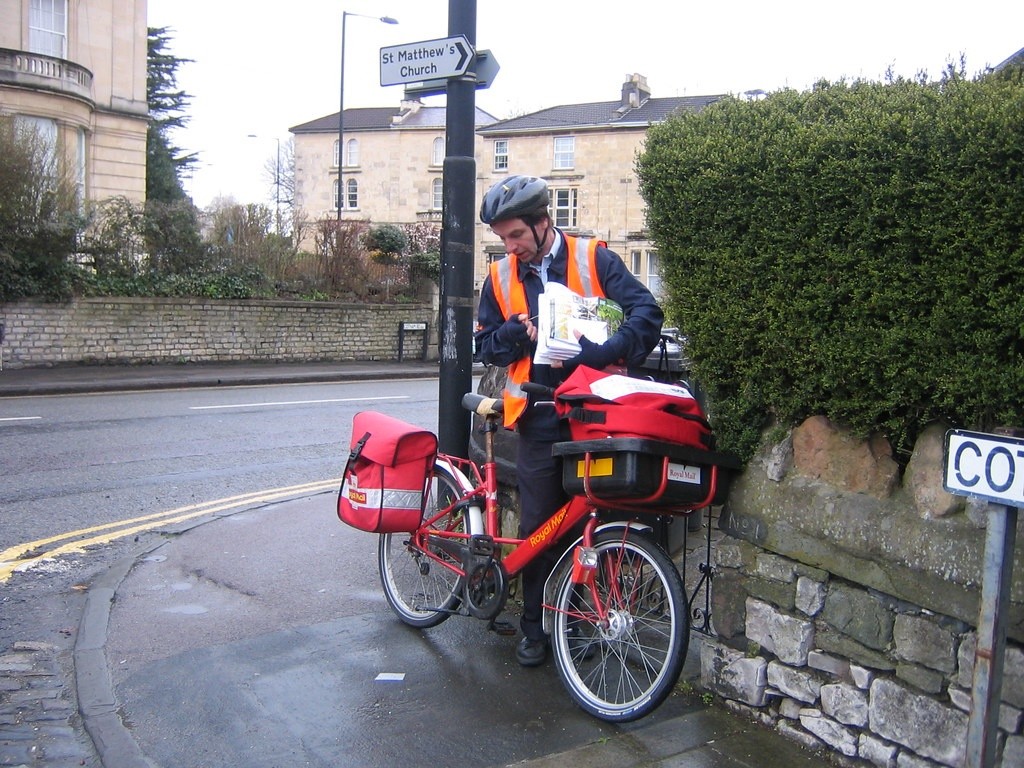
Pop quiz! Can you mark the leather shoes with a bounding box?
[515,635,546,665]
[568,628,595,657]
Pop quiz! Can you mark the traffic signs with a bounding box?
[380,34,475,88]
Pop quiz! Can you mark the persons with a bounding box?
[474,174,664,667]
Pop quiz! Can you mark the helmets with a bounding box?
[479,174,549,224]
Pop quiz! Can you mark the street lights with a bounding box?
[247,134,280,235]
[336,11,399,232]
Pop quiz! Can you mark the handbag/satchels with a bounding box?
[337,410,438,533]
[555,365,715,448]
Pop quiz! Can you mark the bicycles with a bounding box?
[378,380,743,725]
